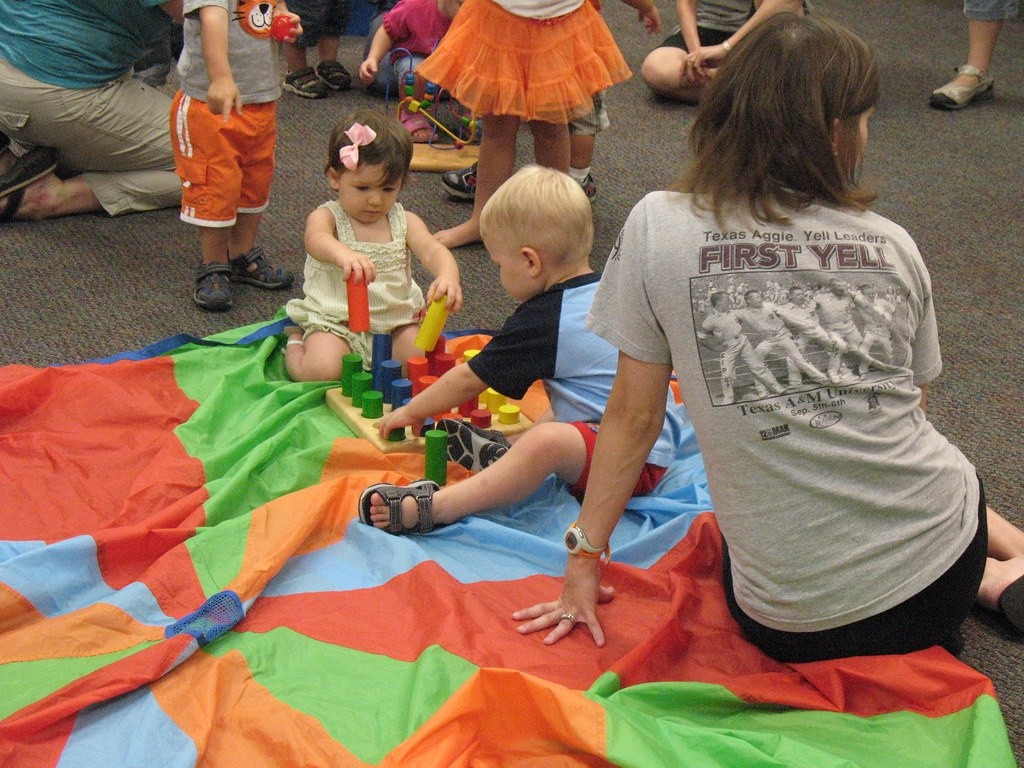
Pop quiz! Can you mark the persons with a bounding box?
[414,0,661,249]
[358,0,466,143]
[283,0,352,99]
[169,0,303,313]
[358,162,684,537]
[641,0,815,103]
[928,0,1024,111]
[0,0,185,222]
[285,110,462,381]
[512,9,1023,665]
[440,86,611,203]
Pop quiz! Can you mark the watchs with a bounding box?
[564,522,611,564]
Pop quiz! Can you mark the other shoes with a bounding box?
[998,562,1024,636]
[930,62,990,108]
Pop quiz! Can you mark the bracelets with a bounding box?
[722,40,731,52]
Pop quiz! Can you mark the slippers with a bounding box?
[0,147,58,218]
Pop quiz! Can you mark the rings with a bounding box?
[560,614,576,628]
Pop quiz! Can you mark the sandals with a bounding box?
[282,325,304,345]
[436,418,512,472]
[226,247,294,290]
[572,172,599,205]
[314,61,351,91]
[358,479,440,533]
[444,161,481,198]
[401,107,440,143]
[283,66,328,99]
[193,260,234,309]
